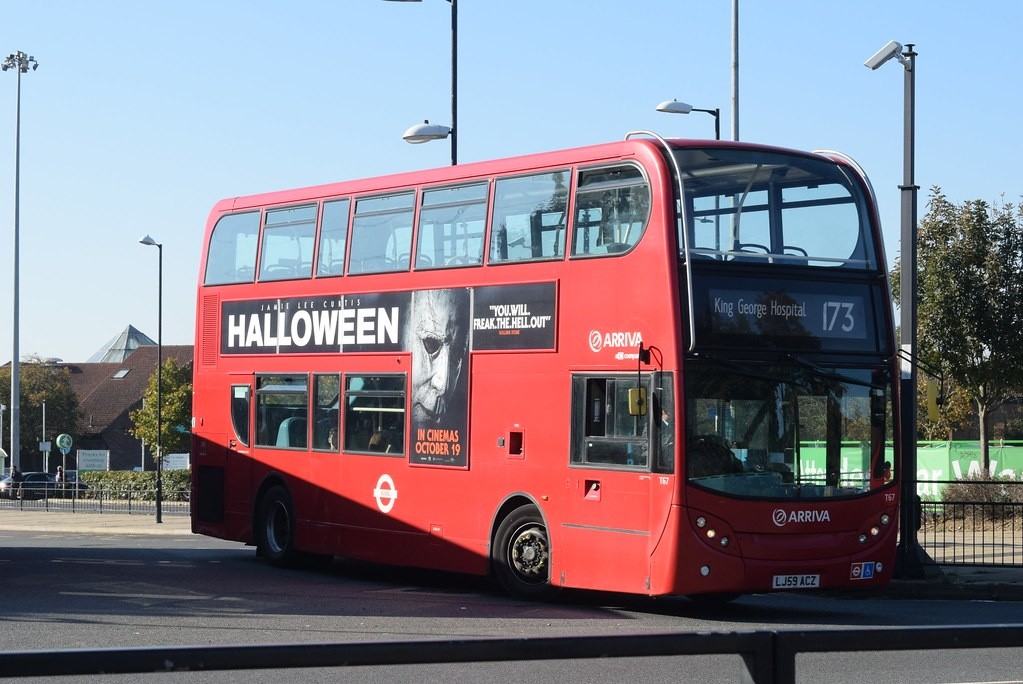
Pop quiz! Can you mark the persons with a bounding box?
[11,465,23,500]
[328,427,340,449]
[401,290,469,467]
[56,467,68,498]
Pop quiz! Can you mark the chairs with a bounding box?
[232,396,403,453]
[237,242,808,283]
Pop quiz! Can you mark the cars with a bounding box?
[0,466,90,500]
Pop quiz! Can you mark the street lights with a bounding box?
[1,48,39,476]
[138,235,162,523]
[401,124,458,257]
[655,98,720,259]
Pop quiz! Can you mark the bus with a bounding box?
[190,130,903,607]
